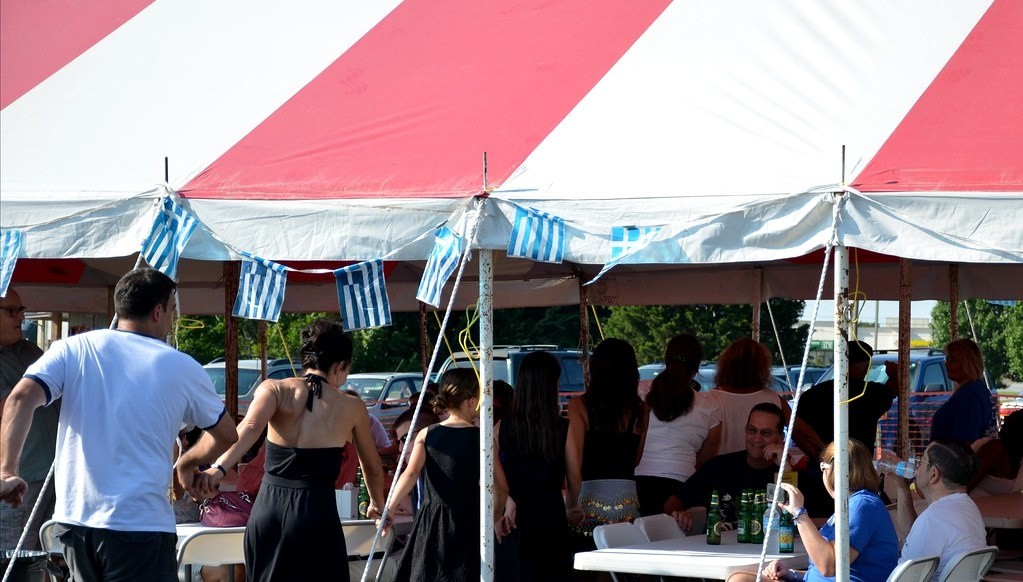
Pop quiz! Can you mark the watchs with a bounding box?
[210,462,227,476]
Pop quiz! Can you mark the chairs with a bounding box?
[38,518,73,582]
[175,507,1000,582]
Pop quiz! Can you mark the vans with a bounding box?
[438,344,592,416]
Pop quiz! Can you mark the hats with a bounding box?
[849,341,873,361]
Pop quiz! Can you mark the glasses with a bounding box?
[0,306,26,317]
[397,430,420,444]
[917,456,929,467]
[820,462,832,472]
[944,356,955,363]
[745,426,779,437]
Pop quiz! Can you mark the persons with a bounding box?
[0,268,1023,582]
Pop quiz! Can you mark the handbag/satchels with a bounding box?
[199,491,256,526]
[725,571,786,582]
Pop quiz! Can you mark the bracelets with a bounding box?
[792,508,808,525]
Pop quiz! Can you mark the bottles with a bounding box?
[737,488,797,553]
[706,489,721,545]
[355,466,370,519]
[877,457,919,479]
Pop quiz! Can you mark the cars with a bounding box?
[770,368,827,391]
[342,373,435,436]
[637,365,799,401]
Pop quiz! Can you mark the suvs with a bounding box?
[204,357,304,415]
[785,348,1000,445]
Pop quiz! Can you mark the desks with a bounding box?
[175,511,414,581]
[972,491,1023,574]
[573,526,809,581]
